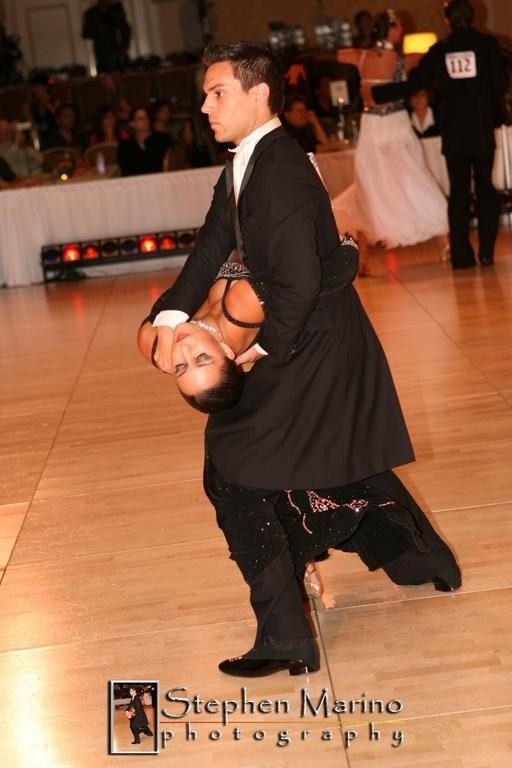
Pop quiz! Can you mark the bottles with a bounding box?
[335,97,354,144]
[95,151,106,175]
[268,16,353,50]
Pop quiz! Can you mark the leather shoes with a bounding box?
[218,644,321,680]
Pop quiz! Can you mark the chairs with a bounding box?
[0,70,218,172]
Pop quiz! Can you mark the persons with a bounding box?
[359,1,510,270]
[148,40,463,678]
[115,684,154,744]
[136,234,434,599]
[334,9,452,277]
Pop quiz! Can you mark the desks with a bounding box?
[0,127,512,287]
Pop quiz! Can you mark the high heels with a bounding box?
[437,240,454,264]
[356,253,370,278]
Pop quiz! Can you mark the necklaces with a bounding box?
[188,319,221,344]
